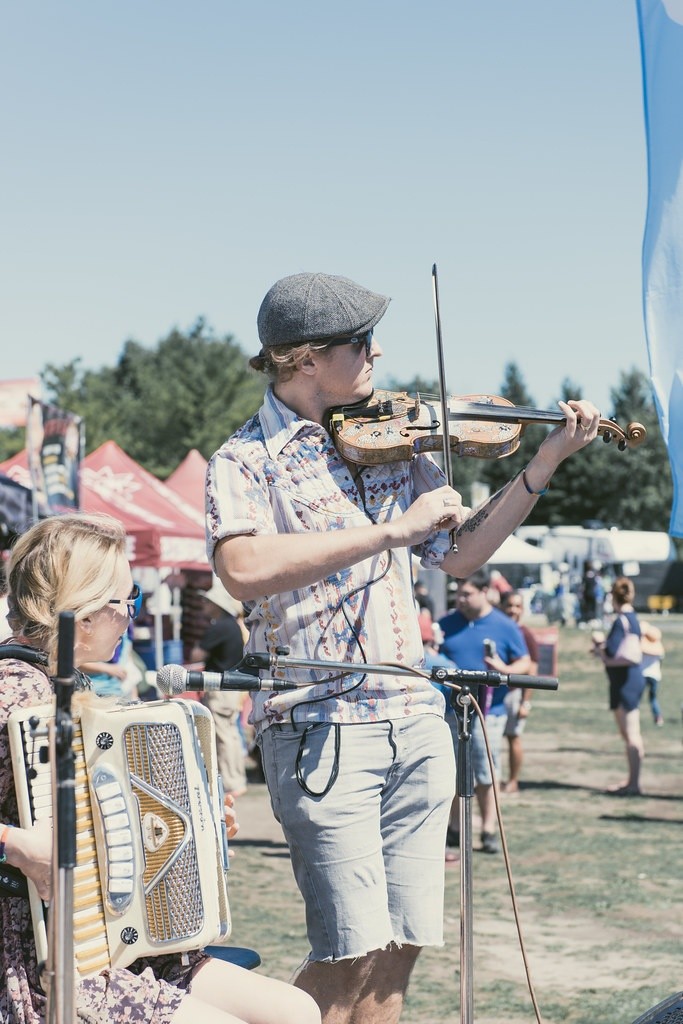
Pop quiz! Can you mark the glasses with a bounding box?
[321,327,373,356]
[109,583,142,619]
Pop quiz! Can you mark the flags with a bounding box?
[24,393,87,525]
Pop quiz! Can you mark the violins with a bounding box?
[330,389,646,467]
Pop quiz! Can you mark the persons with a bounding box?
[0,513,321,1024]
[583,572,664,795]
[414,570,541,853]
[207,273,599,1024]
[189,586,252,799]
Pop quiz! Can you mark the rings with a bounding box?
[580,423,590,431]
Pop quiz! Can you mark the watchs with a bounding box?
[521,701,532,710]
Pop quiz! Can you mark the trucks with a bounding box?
[475,522,683,615]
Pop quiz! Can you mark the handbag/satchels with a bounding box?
[596,613,643,667]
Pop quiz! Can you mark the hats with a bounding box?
[636,620,666,656]
[418,615,435,642]
[256,273,392,346]
[198,585,243,618]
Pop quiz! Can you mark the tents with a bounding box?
[0,439,215,670]
[485,524,683,613]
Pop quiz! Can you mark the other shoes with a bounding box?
[445,833,460,862]
[482,831,499,853]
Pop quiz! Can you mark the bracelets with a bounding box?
[522,465,550,495]
[0,825,14,864]
